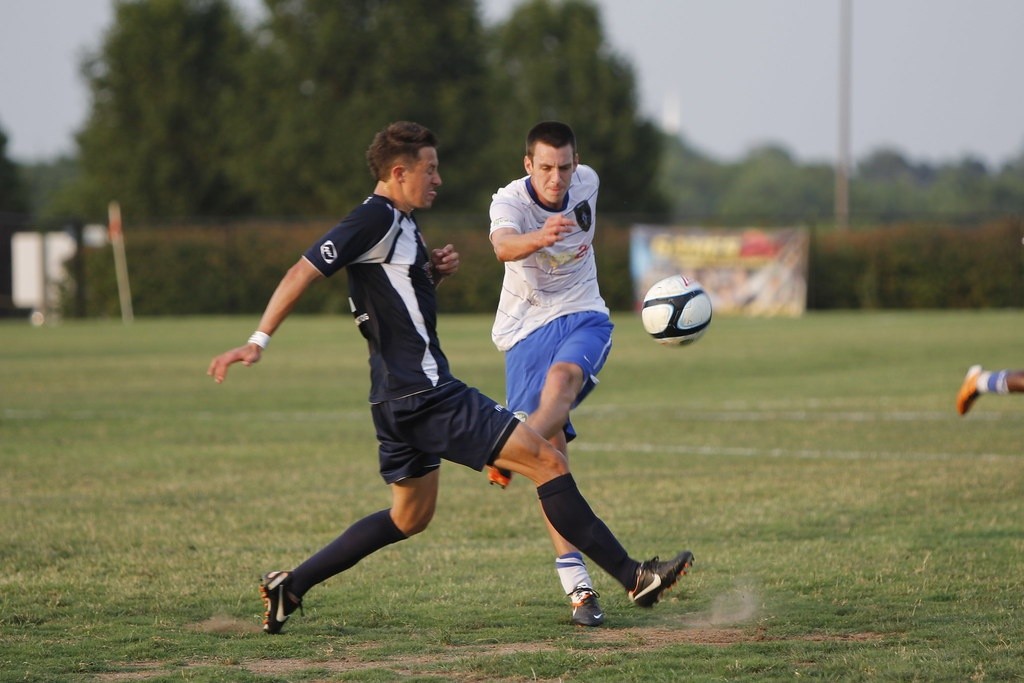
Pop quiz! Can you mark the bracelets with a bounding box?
[248,330,271,350]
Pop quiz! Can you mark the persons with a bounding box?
[956,363,1024,417]
[207,120,697,634]
[489,122,614,627]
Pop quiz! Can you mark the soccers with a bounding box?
[640,274,713,350]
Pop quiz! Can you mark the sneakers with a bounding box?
[568,583,603,626]
[958,364,984,416]
[627,551,696,609]
[260,570,303,634]
[489,465,512,489]
